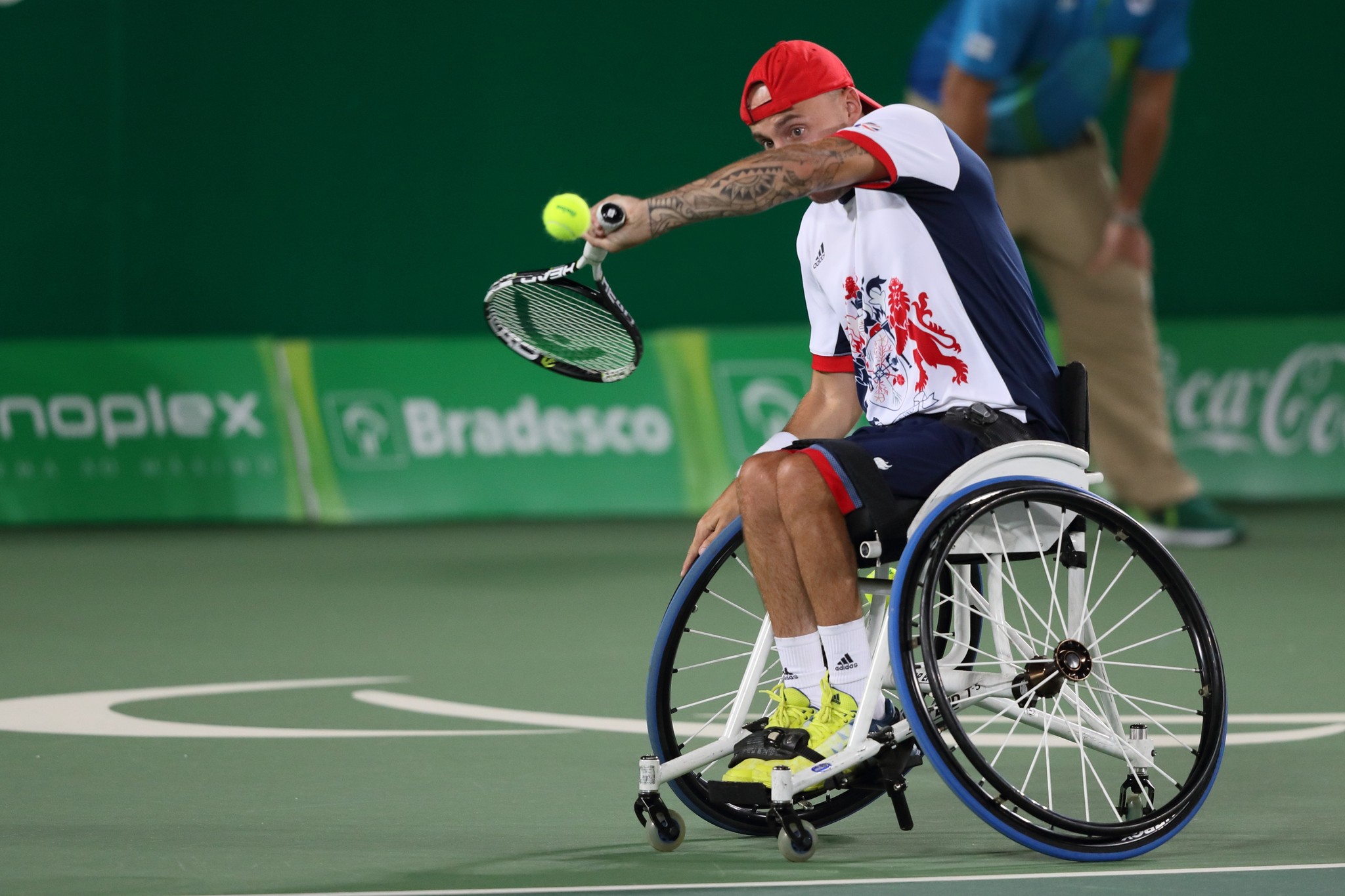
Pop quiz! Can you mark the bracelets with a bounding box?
[731,432,800,481]
[1108,211,1143,227]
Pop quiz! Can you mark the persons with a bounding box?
[580,41,1074,796]
[904,0,1242,551]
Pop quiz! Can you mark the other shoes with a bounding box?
[1117,493,1240,548]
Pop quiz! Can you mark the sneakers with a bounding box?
[750,669,914,793]
[720,682,821,783]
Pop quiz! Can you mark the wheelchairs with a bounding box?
[633,360,1228,862]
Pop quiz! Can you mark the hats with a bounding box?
[740,39,884,126]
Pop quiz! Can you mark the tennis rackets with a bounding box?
[482,201,645,384]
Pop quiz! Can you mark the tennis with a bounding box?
[542,193,592,240]
